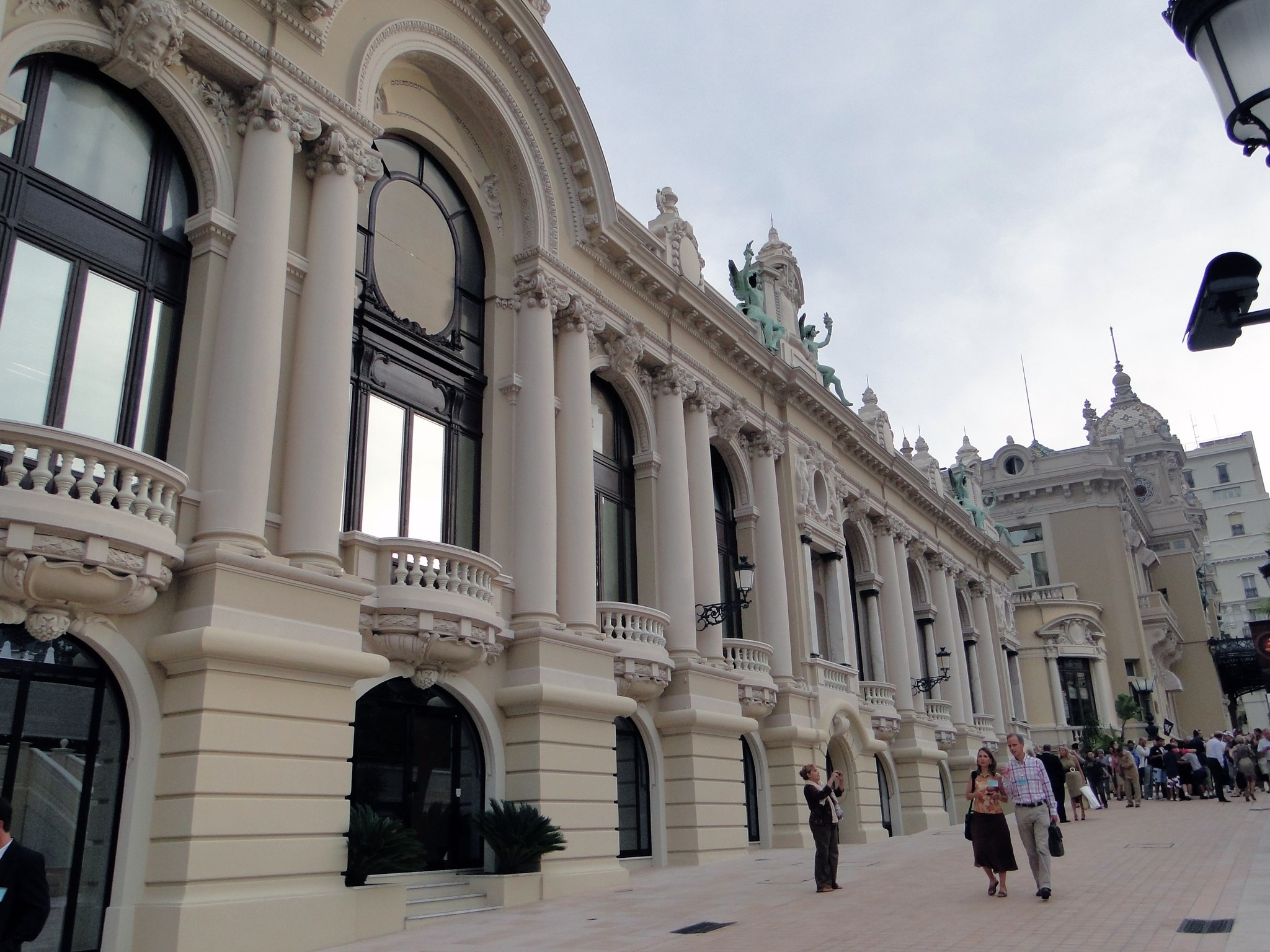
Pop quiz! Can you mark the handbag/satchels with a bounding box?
[1048,819,1065,856]
[965,813,973,841]
[1079,783,1101,810]
[1081,794,1089,810]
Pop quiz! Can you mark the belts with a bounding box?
[1065,767,1079,774]
[1016,799,1047,808]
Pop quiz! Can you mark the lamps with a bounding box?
[733,555,757,607]
[935,646,952,681]
[1132,677,1167,741]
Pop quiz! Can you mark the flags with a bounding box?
[1163,718,1175,736]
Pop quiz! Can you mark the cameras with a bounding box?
[986,791,994,796]
[836,771,841,777]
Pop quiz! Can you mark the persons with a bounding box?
[1058,746,1086,820]
[802,311,852,406]
[1096,749,1111,801]
[1036,744,1071,823]
[1069,743,1088,808]
[1106,728,1270,802]
[742,240,787,351]
[1084,750,1108,810]
[1115,746,1141,807]
[1000,732,1059,900]
[800,764,846,894]
[956,461,986,531]
[982,487,1020,547]
[965,747,1018,897]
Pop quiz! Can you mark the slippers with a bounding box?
[816,885,843,893]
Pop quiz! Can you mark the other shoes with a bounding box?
[988,881,999,895]
[1082,815,1085,820]
[1103,785,1270,808]
[1036,888,1051,899]
[1060,820,1071,823]
[1074,815,1078,820]
[997,889,1007,897]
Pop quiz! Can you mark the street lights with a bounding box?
[1131,675,1162,741]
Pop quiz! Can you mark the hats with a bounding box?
[1222,731,1233,737]
[1233,728,1237,733]
